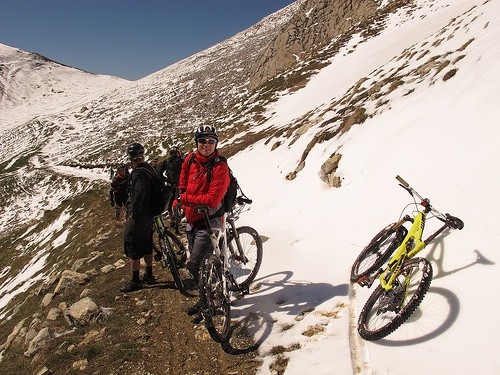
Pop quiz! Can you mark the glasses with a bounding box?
[197,138,216,145]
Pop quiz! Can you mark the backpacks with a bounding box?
[186,155,240,212]
[164,157,184,192]
[134,165,165,218]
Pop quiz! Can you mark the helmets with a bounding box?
[170,146,180,154]
[117,164,125,171]
[126,143,144,160]
[194,125,219,142]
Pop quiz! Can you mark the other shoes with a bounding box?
[119,280,143,292]
[115,220,122,226]
[124,218,129,225]
[140,276,155,288]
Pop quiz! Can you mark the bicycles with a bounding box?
[184,193,264,344]
[349,174,465,342]
[150,208,188,295]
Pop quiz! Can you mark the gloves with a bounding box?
[109,201,115,206]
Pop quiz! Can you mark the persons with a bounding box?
[108,164,132,222]
[118,142,168,293]
[154,144,184,228]
[172,124,239,324]
